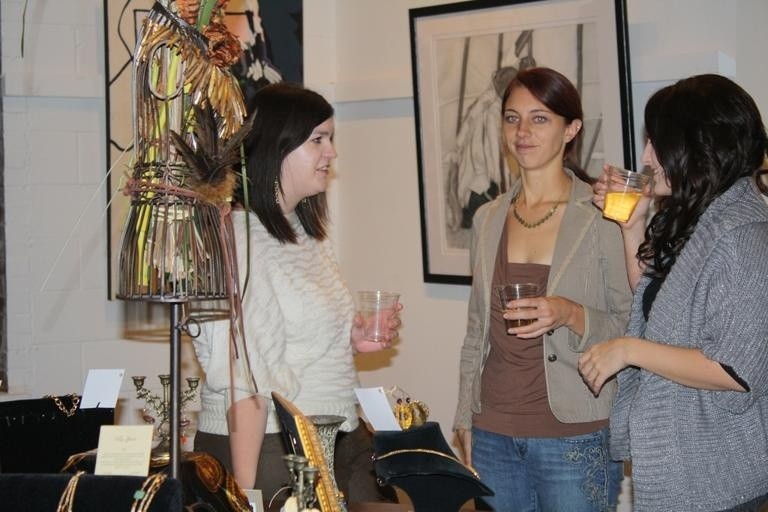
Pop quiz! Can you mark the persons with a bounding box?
[187,82,405,512]
[449,64,635,512]
[576,72,768,510]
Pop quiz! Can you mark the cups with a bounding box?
[496,281,540,338]
[355,289,402,344]
[600,162,651,225]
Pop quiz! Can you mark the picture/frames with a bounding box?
[407,1,638,287]
[269,385,347,512]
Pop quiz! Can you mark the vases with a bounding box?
[302,410,347,512]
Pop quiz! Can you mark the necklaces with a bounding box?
[513,189,564,229]
[57,470,170,511]
[370,448,481,482]
[42,392,80,417]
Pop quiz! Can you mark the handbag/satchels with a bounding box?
[369,421,495,509]
